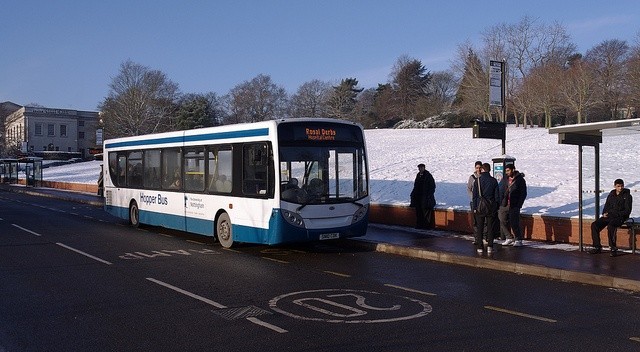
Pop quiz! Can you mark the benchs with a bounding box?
[608,217,640,255]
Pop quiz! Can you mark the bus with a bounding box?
[100,116,372,249]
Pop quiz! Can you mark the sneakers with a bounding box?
[487,247,495,254]
[592,248,603,255]
[472,240,476,245]
[514,241,524,247]
[502,238,514,246]
[477,248,483,254]
[609,247,618,256]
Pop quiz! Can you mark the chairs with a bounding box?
[280,180,289,192]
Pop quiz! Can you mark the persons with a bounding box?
[472,163,499,254]
[468,161,488,245]
[169,179,181,189]
[110,160,142,176]
[410,164,436,230]
[499,164,527,246]
[590,179,632,257]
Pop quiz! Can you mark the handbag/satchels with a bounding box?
[501,176,517,207]
[477,176,497,217]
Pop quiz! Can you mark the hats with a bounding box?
[417,164,426,168]
